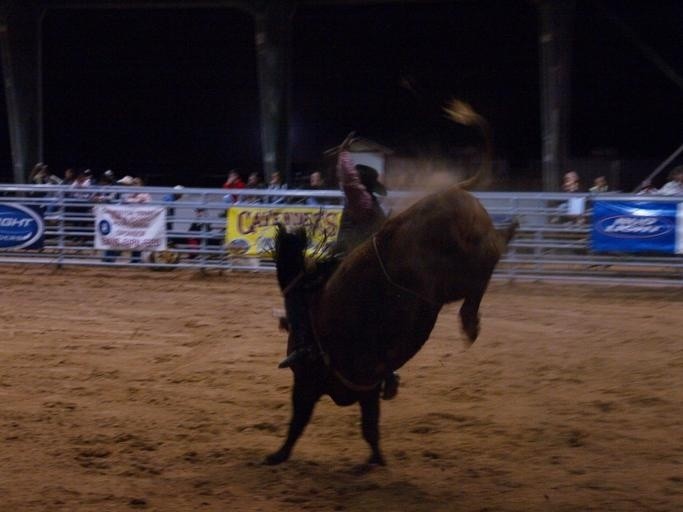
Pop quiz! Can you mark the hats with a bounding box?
[354,163,388,196]
[28,162,190,200]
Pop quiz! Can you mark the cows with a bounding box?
[259,98,521,465]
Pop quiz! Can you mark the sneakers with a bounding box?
[382,372,400,400]
[278,348,309,369]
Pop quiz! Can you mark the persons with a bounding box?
[658,163,682,196]
[635,179,658,195]
[551,170,593,270]
[589,175,616,270]
[290,166,332,204]
[279,130,402,401]
[29,164,290,265]
